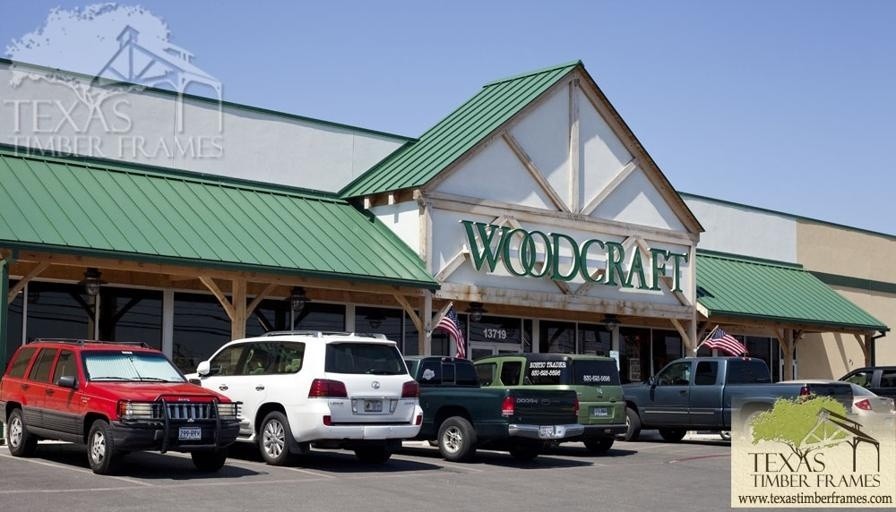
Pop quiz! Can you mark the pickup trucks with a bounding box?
[615,355,854,444]
[377,353,585,463]
[837,365,895,399]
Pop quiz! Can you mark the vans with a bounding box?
[471,351,629,452]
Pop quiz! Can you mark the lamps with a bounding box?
[284,286,311,311]
[464,303,488,322]
[78,268,108,295]
[599,314,621,331]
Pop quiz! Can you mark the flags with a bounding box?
[703,327,749,357]
[435,306,466,359]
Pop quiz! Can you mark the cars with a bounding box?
[721,376,896,443]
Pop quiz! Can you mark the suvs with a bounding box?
[1,335,243,476]
[183,327,425,465]
